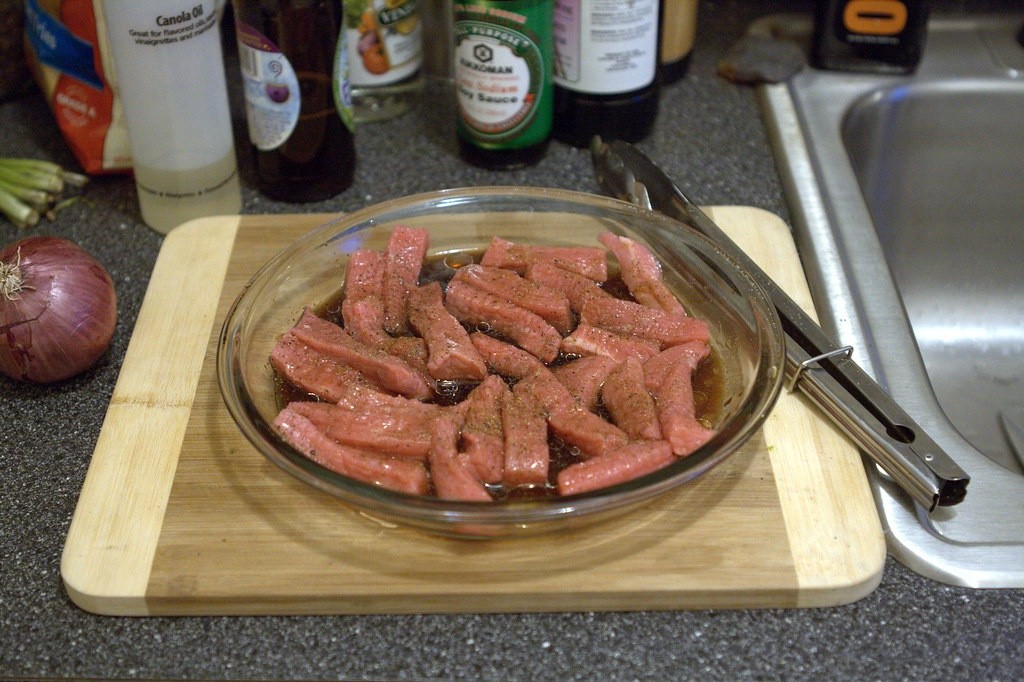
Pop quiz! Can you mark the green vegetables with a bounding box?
[0,158,88,227]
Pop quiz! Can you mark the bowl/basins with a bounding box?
[216,184,785,538]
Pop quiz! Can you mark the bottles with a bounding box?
[555,0,666,149]
[452,0,554,169]
[234,1,355,203]
[343,0,429,126]
[101,0,242,233]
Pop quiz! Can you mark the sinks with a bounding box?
[754,72,1024,590]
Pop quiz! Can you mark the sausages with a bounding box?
[266,224,710,533]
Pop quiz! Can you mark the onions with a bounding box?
[0,235,116,383]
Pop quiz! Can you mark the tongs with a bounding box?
[590,135,970,513]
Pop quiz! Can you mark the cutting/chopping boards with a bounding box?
[59,207,886,612]
